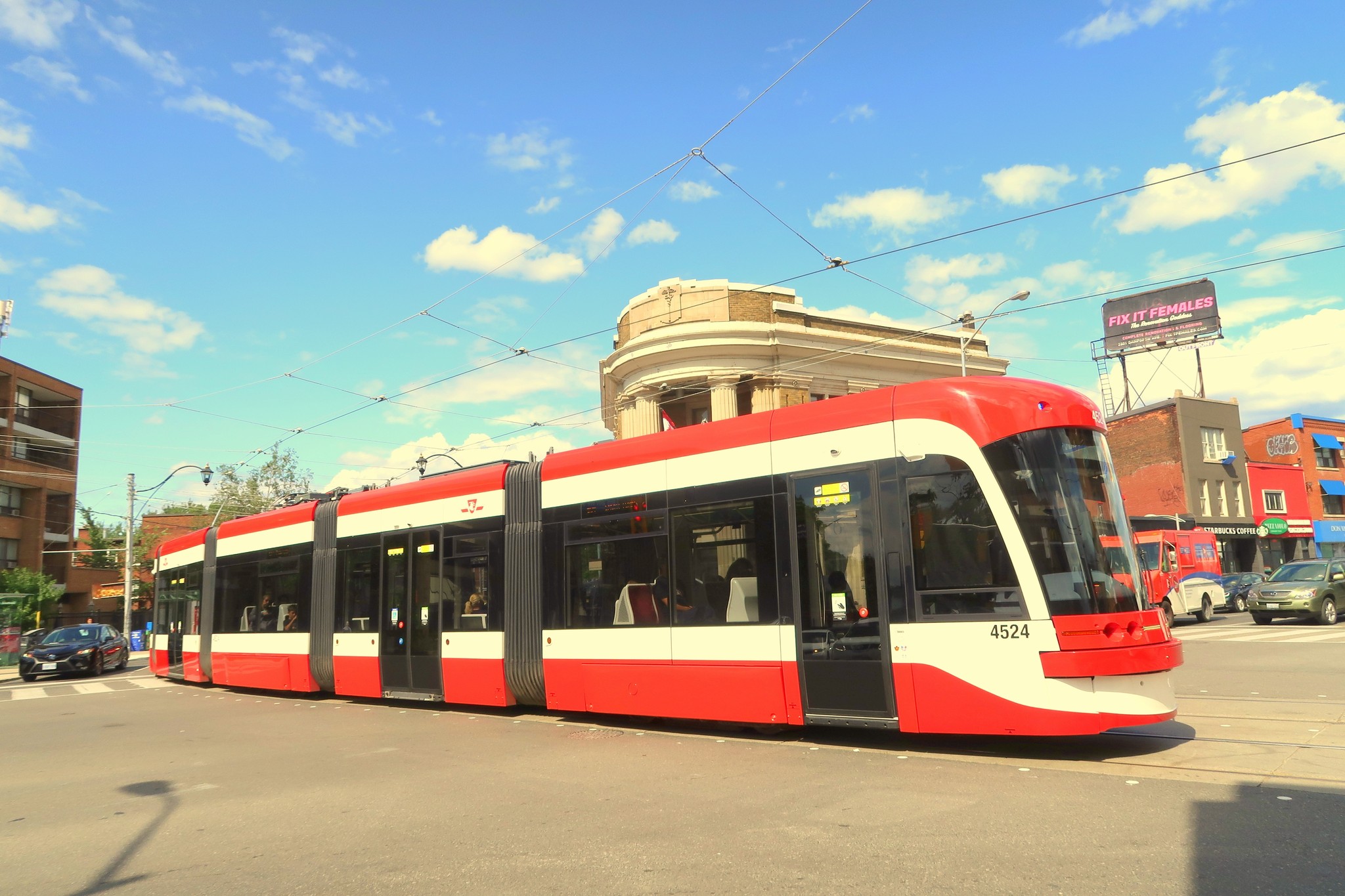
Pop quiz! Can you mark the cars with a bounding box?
[19,623,128,683]
[1221,572,1270,612]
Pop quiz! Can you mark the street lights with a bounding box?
[960,291,1031,376]
[124,464,214,659]
[1144,513,1185,530]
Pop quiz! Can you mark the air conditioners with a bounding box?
[6,561,17,568]
[16,407,30,418]
[11,509,20,515]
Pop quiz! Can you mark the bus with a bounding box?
[149,376,1183,739]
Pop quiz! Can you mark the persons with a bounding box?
[569,522,777,626]
[248,593,289,632]
[464,592,493,630]
[87,617,93,624]
[822,572,860,624]
[283,604,298,631]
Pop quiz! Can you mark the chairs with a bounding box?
[703,573,728,613]
[459,614,488,630]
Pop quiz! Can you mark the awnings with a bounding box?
[1318,480,1345,496]
[1311,433,1344,450]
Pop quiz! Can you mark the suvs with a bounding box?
[1246,557,1345,625]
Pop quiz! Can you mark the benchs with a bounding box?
[612,577,667,625]
[351,617,369,631]
[239,603,299,631]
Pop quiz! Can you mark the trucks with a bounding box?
[1132,530,1226,628]
